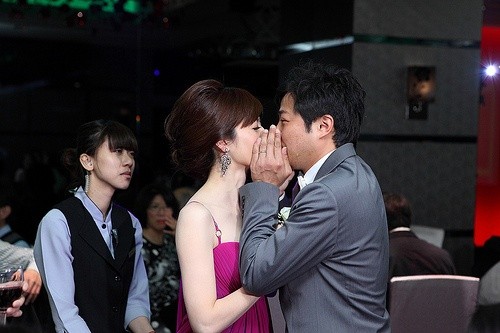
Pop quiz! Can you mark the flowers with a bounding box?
[279,206,290,226]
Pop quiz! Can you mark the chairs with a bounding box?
[388,274,480,333]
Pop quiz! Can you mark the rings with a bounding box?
[260,151,267,153]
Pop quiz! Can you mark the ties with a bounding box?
[291,182,300,203]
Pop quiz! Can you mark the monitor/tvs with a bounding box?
[410,226,445,250]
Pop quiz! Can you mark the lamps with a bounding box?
[409,64,438,122]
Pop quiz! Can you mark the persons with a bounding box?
[32,119,160,333]
[237,58,390,333]
[165,79,274,333]
[0,171,500,333]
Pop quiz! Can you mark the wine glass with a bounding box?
[0,262,24,327]
